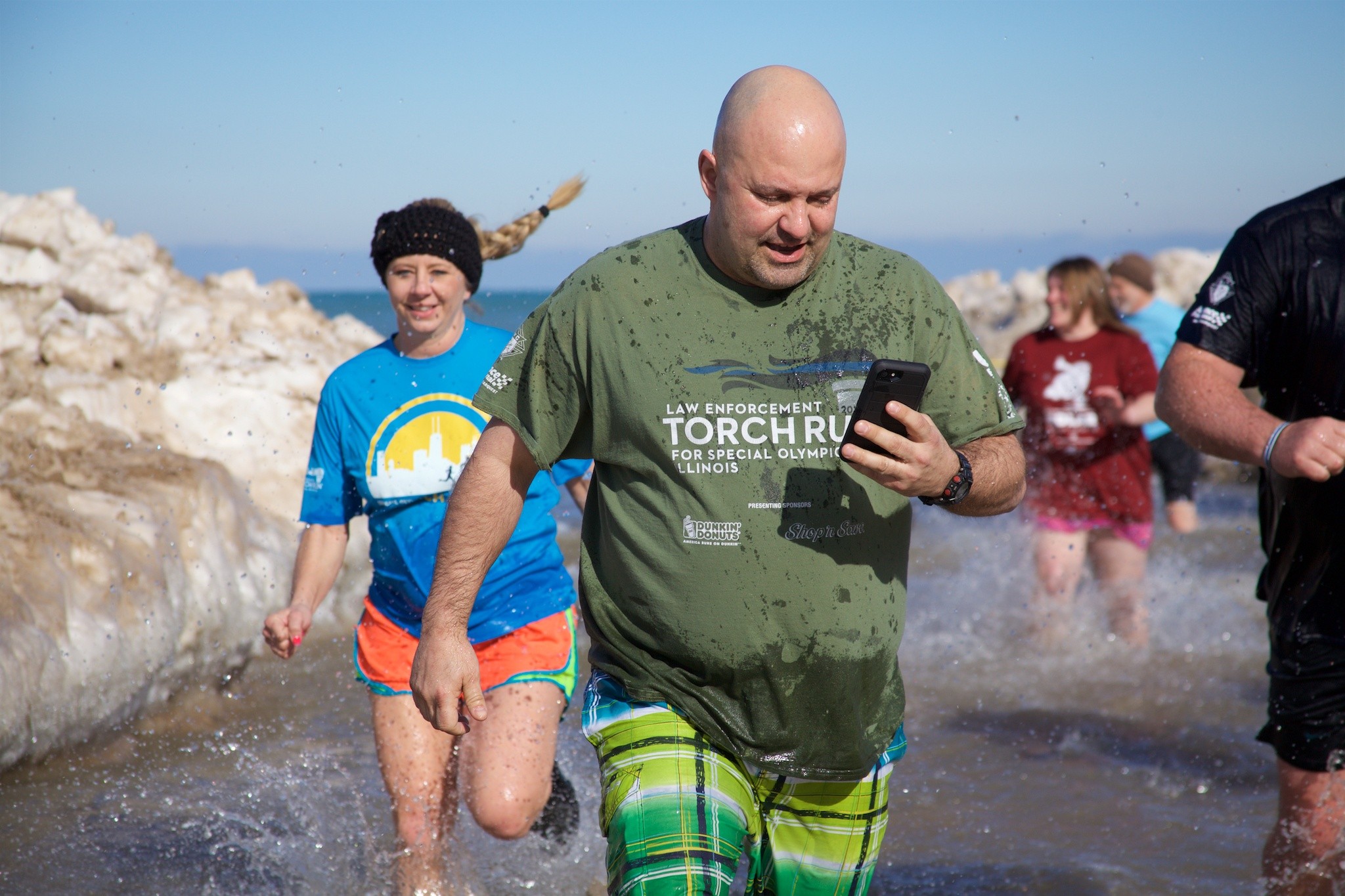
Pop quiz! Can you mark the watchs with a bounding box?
[917,450,974,506]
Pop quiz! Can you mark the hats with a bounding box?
[1110,254,1153,291]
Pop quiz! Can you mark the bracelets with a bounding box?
[1264,423,1292,469]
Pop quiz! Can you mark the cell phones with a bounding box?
[837,359,930,464]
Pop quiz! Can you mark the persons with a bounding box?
[1000,257,1163,652]
[407,65,1028,894]
[1105,251,1201,534]
[263,177,595,896]
[1154,177,1345,896]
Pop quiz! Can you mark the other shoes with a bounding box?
[529,760,581,856]
